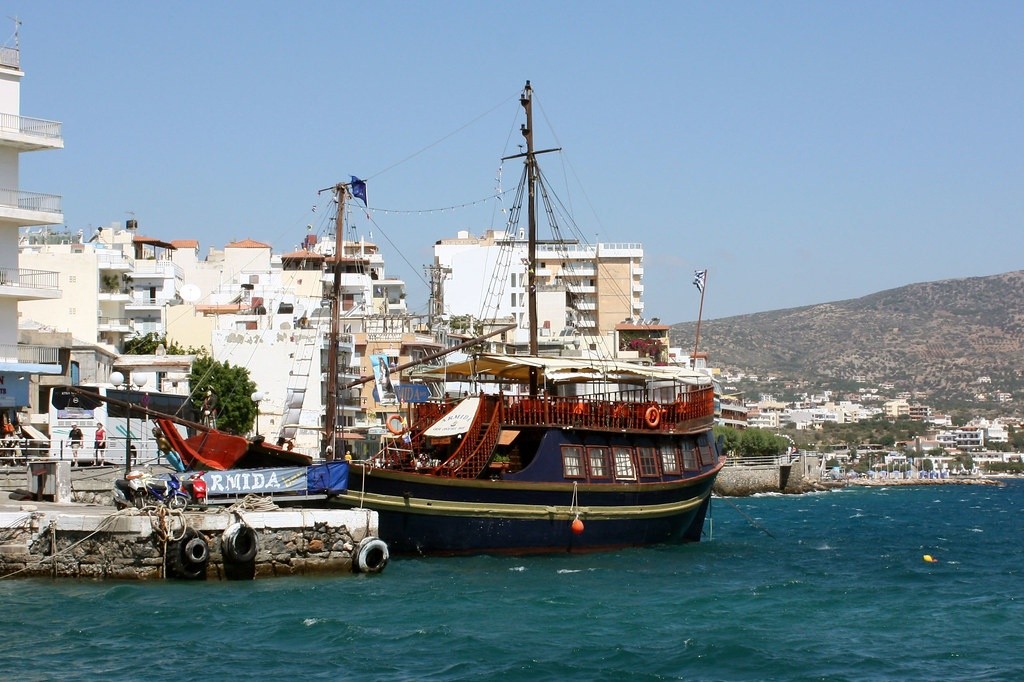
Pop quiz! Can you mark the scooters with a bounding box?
[177,469,208,513]
[111,469,147,510]
[144,471,188,514]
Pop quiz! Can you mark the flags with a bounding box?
[350,172,370,206]
[691,269,706,293]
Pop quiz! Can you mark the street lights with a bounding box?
[110,369,147,472]
[251,391,264,436]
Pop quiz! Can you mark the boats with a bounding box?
[155,75,724,558]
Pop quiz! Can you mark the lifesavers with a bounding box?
[385,414,406,435]
[645,406,661,428]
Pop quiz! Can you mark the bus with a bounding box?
[47,384,202,466]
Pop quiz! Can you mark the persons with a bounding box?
[65,423,85,471]
[92,422,106,468]
[344,450,353,463]
[201,386,220,428]
